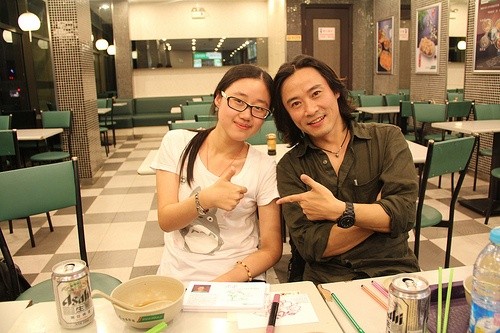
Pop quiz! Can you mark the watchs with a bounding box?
[336,201,355,228]
[195,192,209,216]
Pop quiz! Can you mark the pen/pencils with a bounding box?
[330,292,364,333]
[360,285,388,310]
[371,280,388,298]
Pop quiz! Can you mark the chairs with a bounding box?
[0,90,500,303]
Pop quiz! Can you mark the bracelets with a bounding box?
[236,261,252,282]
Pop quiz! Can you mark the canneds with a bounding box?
[385,274,432,333]
[52,259,95,330]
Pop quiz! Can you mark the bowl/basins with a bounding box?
[109,275,186,329]
[463,272,473,305]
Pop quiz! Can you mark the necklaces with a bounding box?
[206,137,245,177]
[310,128,348,158]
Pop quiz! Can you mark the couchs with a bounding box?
[100,98,134,130]
[132,95,210,128]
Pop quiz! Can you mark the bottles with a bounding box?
[465,226,500,333]
[267,133,277,155]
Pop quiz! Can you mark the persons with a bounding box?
[150,63,283,286]
[194,287,207,293]
[270,54,420,286]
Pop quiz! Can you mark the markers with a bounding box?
[266,294,280,333]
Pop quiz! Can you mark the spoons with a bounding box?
[90,289,174,312]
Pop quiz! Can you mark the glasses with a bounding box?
[220,91,272,120]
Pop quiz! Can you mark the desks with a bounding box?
[357,105,408,135]
[0,128,65,165]
[98,107,112,152]
[138,143,294,177]
[431,119,500,216]
[0,265,474,333]
